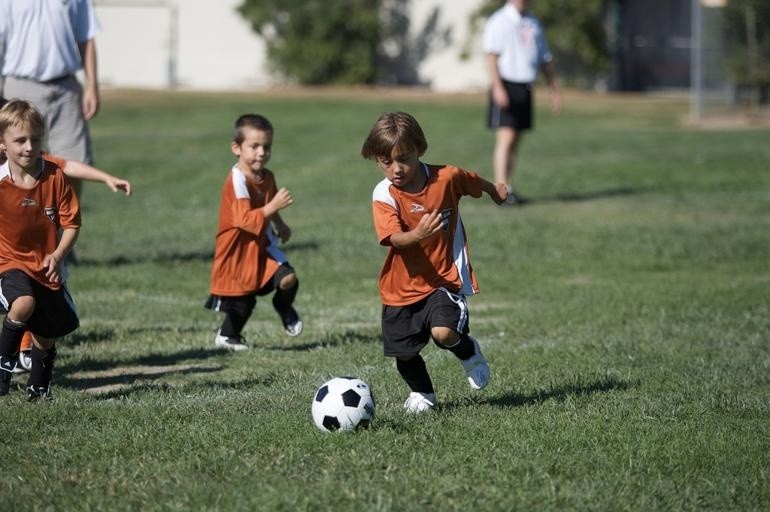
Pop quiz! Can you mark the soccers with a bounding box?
[311,375,376,433]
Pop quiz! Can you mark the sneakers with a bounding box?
[405,392,438,414]
[215,329,249,352]
[12,348,34,373]
[459,337,489,390]
[26,377,52,403]
[0,356,20,395]
[273,296,303,338]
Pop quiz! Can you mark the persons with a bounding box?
[1,0,102,266]
[1,149,133,377]
[205,113,305,353]
[481,0,562,208]
[358,111,510,418]
[1,98,81,403]
